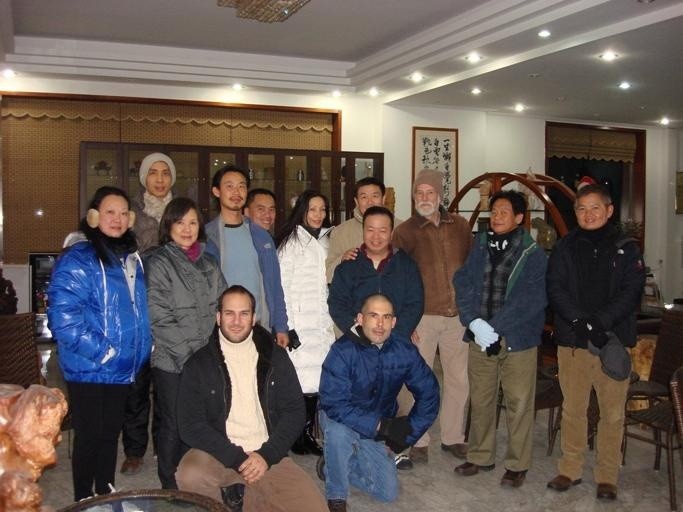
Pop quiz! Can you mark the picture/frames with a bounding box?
[675,172,683,214]
[412,126,458,216]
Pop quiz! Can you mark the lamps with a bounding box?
[216,0,310,23]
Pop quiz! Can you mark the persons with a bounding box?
[327,205,426,470]
[451,187,549,488]
[199,166,290,510]
[240,187,280,249]
[315,291,441,511]
[390,167,475,469]
[175,285,330,511]
[45,185,154,500]
[120,153,171,477]
[325,173,387,341]
[544,174,646,502]
[276,188,335,456]
[142,197,230,489]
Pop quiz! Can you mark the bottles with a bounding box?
[297,168,304,181]
[249,168,254,180]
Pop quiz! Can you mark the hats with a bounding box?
[412,169,444,200]
[139,152,177,189]
[589,331,631,381]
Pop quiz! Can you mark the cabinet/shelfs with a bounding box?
[78,141,384,235]
[448,173,576,253]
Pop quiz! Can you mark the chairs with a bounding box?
[0,313,41,388]
[54,490,232,512]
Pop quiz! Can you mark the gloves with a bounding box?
[469,319,499,345]
